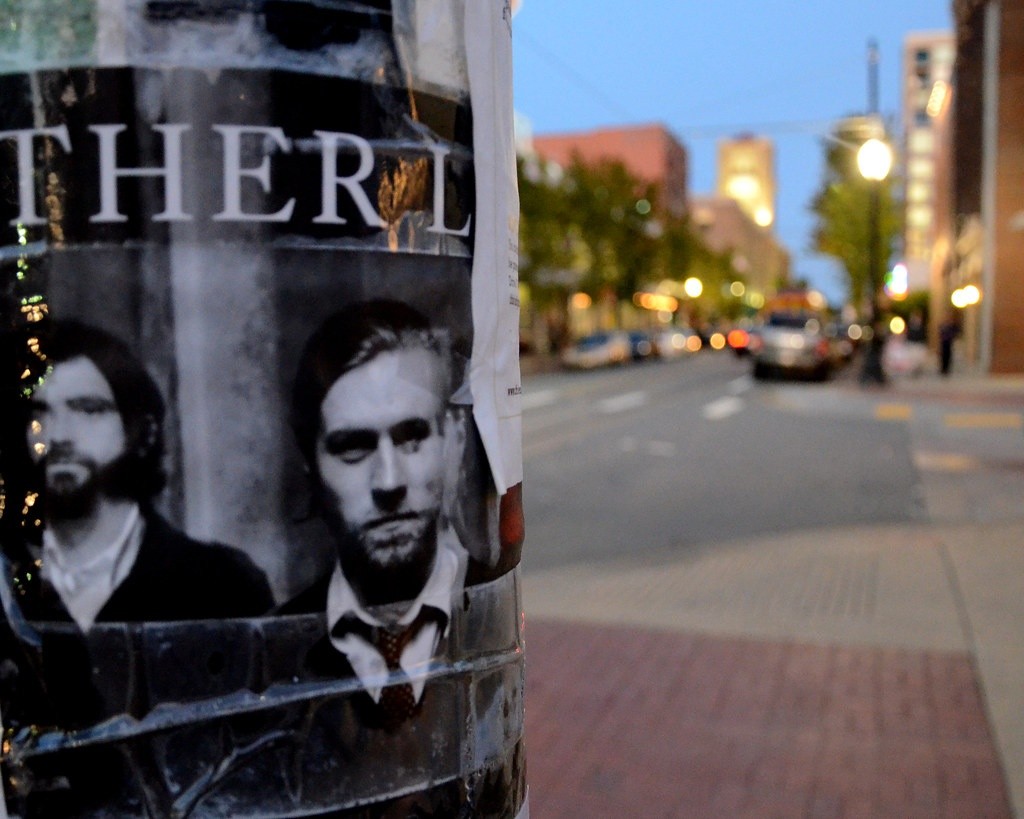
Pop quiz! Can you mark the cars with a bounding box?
[558,325,728,371]
[750,308,853,383]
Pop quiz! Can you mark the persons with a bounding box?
[275,295,497,733]
[937,311,959,373]
[858,316,887,387]
[0,319,275,725]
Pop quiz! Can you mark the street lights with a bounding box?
[855,138,897,386]
